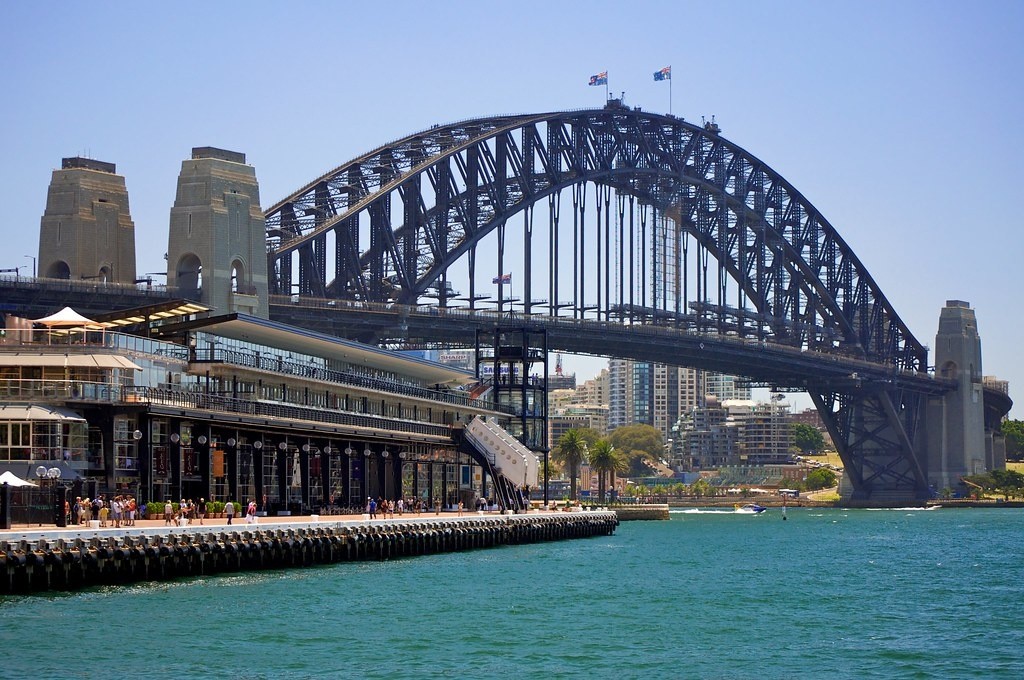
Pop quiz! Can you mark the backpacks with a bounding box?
[73,502,80,513]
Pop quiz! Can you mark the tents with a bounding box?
[25,306,108,347]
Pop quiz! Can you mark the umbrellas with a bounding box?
[0,471,40,488]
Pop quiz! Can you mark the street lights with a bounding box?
[618,487,622,507]
[48,467,62,525]
[35,465,47,527]
[636,485,639,505]
[609,486,613,508]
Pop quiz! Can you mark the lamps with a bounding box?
[148,433,180,445]
[306,446,331,456]
[235,441,262,451]
[113,430,142,443]
[209,438,236,449]
[386,452,406,461]
[369,451,389,460]
[262,442,287,452]
[180,435,207,447]
[286,444,310,454]
[349,449,371,458]
[328,448,352,456]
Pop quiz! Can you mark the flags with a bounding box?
[653,65,671,81]
[589,71,608,86]
[493,274,510,284]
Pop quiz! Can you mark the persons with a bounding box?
[470,495,529,514]
[458,500,464,516]
[552,498,558,512]
[165,498,207,526]
[564,498,570,511]
[223,499,234,525]
[65,494,136,528]
[368,495,442,519]
[246,498,257,523]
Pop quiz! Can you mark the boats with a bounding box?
[735,504,766,514]
[529,479,573,500]
[924,506,942,511]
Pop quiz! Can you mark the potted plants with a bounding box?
[565,501,571,512]
[220,502,227,518]
[205,502,215,518]
[155,502,164,520]
[162,503,174,519]
[145,501,157,520]
[171,503,179,519]
[232,501,242,518]
[213,500,221,518]
[277,504,292,516]
[256,502,267,517]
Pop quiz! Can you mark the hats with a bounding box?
[188,499,192,502]
[200,498,204,500]
[167,500,171,503]
[181,499,185,502]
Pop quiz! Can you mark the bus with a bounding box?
[778,488,799,497]
